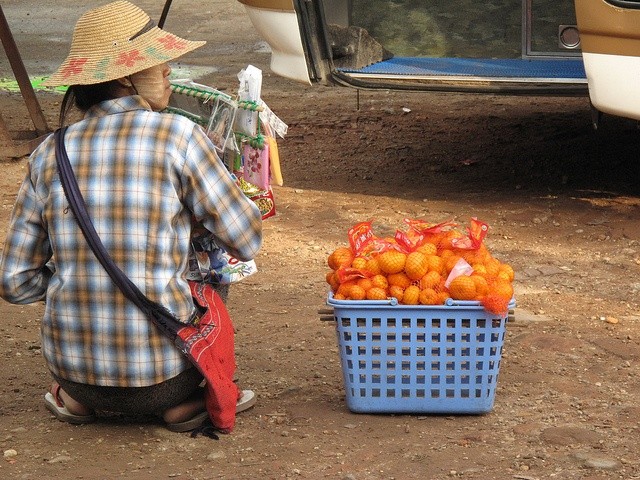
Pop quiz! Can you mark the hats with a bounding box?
[37,0,206,86]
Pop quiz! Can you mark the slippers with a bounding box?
[43,380,97,425]
[165,388,256,433]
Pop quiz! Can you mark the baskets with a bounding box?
[327,291,515,416]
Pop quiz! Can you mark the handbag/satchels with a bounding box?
[55,126,242,433]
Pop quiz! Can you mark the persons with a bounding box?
[0,1,263,432]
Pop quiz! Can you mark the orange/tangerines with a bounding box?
[326,220,514,315]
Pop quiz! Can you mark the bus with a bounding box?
[238,0,640,122]
